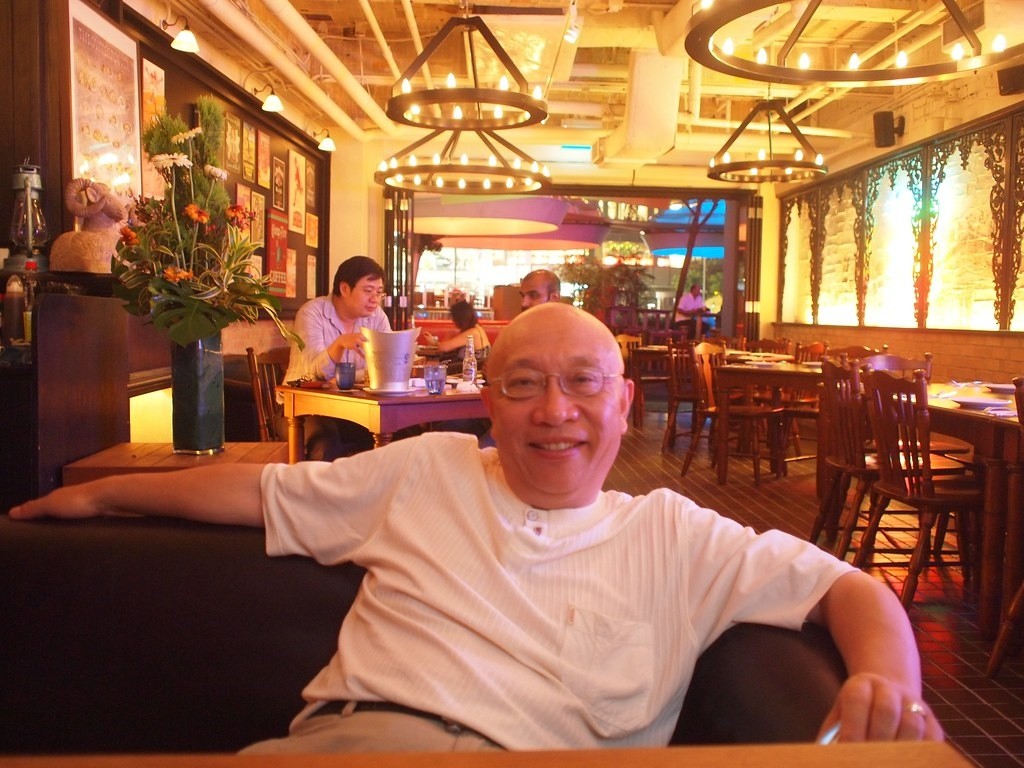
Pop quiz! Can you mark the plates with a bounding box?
[745,361,780,368]
[362,388,417,396]
[950,397,1013,410]
[801,361,822,367]
[984,383,1016,394]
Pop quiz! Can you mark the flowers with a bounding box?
[112,92,308,354]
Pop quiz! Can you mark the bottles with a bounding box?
[19,262,40,310]
[462,335,478,384]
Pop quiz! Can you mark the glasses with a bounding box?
[491,369,627,400]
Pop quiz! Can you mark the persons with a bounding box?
[272,255,392,459]
[10,301,943,755]
[519,269,560,311]
[675,284,710,339]
[421,301,491,376]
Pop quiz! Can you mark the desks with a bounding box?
[65,444,287,488]
[3,740,969,768]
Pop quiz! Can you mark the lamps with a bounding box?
[162,14,199,52]
[374,124,554,197]
[707,52,829,185]
[874,110,905,147]
[253,84,285,111]
[383,0,548,130]
[998,64,1024,95]
[10,158,52,273]
[314,128,335,151]
[684,0,1006,88]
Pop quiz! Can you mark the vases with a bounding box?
[171,319,228,455]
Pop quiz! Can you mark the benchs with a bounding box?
[0,518,838,755]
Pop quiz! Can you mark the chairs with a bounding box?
[245,344,294,445]
[588,305,1024,678]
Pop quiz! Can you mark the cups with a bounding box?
[335,362,356,390]
[423,365,447,396]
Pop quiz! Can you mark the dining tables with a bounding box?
[631,344,745,429]
[814,383,1024,643]
[414,345,440,358]
[711,360,873,487]
[694,312,717,341]
[274,377,490,464]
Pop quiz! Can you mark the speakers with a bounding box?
[997,64,1024,95]
[873,110,895,147]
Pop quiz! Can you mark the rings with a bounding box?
[904,702,926,715]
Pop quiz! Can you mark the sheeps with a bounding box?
[50,178,128,274]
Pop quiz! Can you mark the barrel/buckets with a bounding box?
[360,326,422,389]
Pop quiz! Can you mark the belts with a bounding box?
[304,699,470,732]
